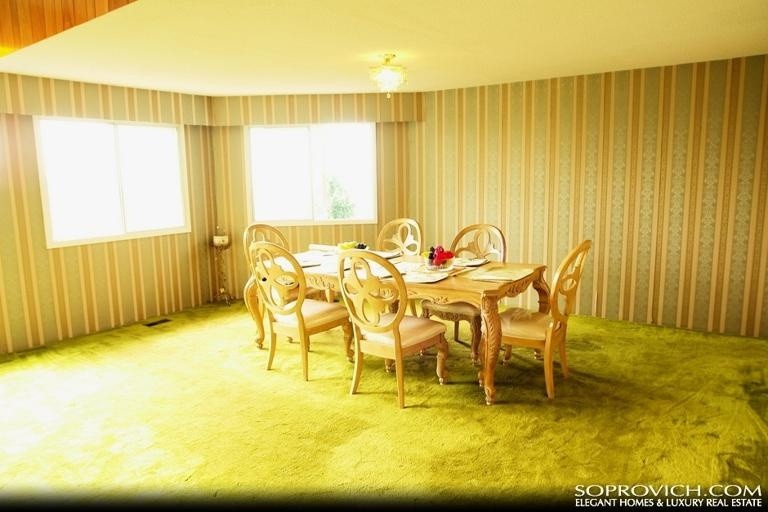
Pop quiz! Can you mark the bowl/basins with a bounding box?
[423,256,455,272]
[337,243,369,257]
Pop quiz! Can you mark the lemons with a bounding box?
[442,258,454,268]
[338,242,357,250]
[422,252,430,259]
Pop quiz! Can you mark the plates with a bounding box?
[296,260,448,285]
[453,258,488,266]
[309,245,338,255]
[370,250,400,258]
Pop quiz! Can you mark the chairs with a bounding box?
[419,225,506,365]
[478,238,592,400]
[244,224,314,349]
[338,251,449,410]
[249,242,355,381]
[376,218,423,317]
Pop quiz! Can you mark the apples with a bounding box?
[432,245,454,266]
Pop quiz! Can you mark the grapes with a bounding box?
[355,244,367,248]
[429,246,434,258]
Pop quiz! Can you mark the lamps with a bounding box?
[370,53,409,99]
